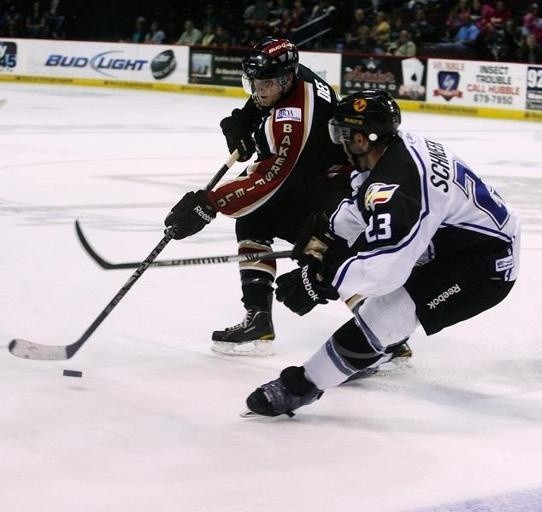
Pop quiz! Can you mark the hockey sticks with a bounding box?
[74,220,294,271]
[8,147,243,361]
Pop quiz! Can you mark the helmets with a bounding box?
[239,35,301,98]
[328,87,403,146]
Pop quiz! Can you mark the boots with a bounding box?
[210,303,276,344]
[245,364,325,418]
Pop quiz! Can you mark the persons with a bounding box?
[165,36,411,384]
[245,88,518,417]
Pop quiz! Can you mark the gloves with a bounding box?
[288,208,337,270]
[220,107,255,163]
[275,263,340,317]
[163,189,218,241]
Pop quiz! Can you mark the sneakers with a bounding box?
[381,335,414,365]
